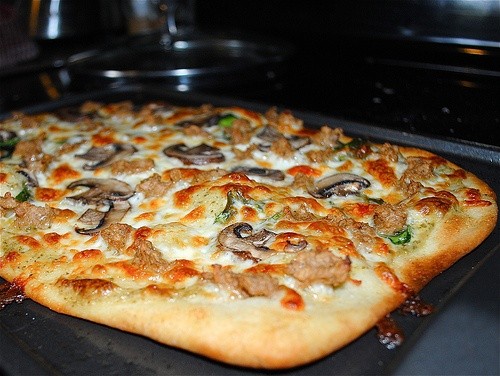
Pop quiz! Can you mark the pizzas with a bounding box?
[0,102,500,368]
